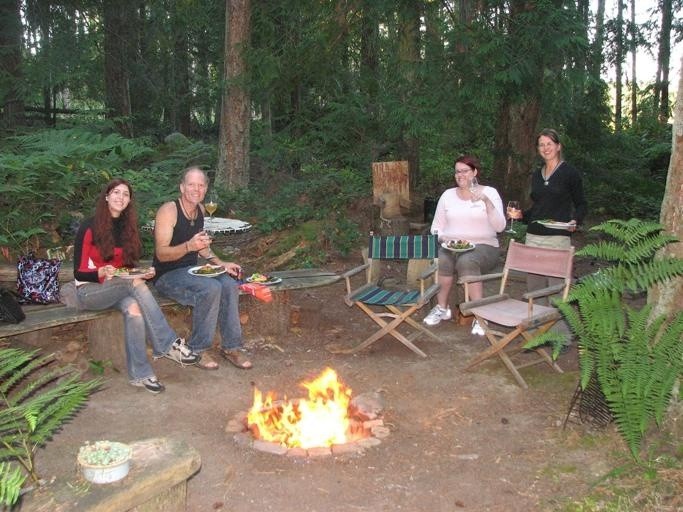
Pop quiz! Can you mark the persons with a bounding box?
[149,164,254,373]
[420,155,509,337]
[505,126,589,355]
[72,177,203,397]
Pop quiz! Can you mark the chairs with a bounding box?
[342,231,443,358]
[458,238,576,390]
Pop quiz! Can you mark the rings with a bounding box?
[236,268,242,273]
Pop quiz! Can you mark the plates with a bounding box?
[246,276,282,286]
[112,269,148,279]
[441,240,475,252]
[186,265,227,277]
[538,221,575,230]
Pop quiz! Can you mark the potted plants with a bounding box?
[521,217,683,461]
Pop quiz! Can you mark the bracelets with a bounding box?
[184,239,191,255]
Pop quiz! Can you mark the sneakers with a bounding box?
[423,304,451,326]
[561,345,570,354]
[165,337,200,366]
[471,319,488,336]
[131,379,164,394]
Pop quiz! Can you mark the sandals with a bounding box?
[220,350,252,369]
[196,351,218,370]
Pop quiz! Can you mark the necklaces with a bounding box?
[543,160,561,187]
[179,197,198,229]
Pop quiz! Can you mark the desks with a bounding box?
[140,217,253,240]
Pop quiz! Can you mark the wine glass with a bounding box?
[200,228,215,260]
[204,194,218,226]
[505,200,519,234]
[467,176,480,208]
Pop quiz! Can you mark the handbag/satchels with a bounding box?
[0,286,26,323]
[16,255,62,303]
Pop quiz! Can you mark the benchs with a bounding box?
[0,268,342,374]
[0,433,203,511]
[489,250,646,299]
